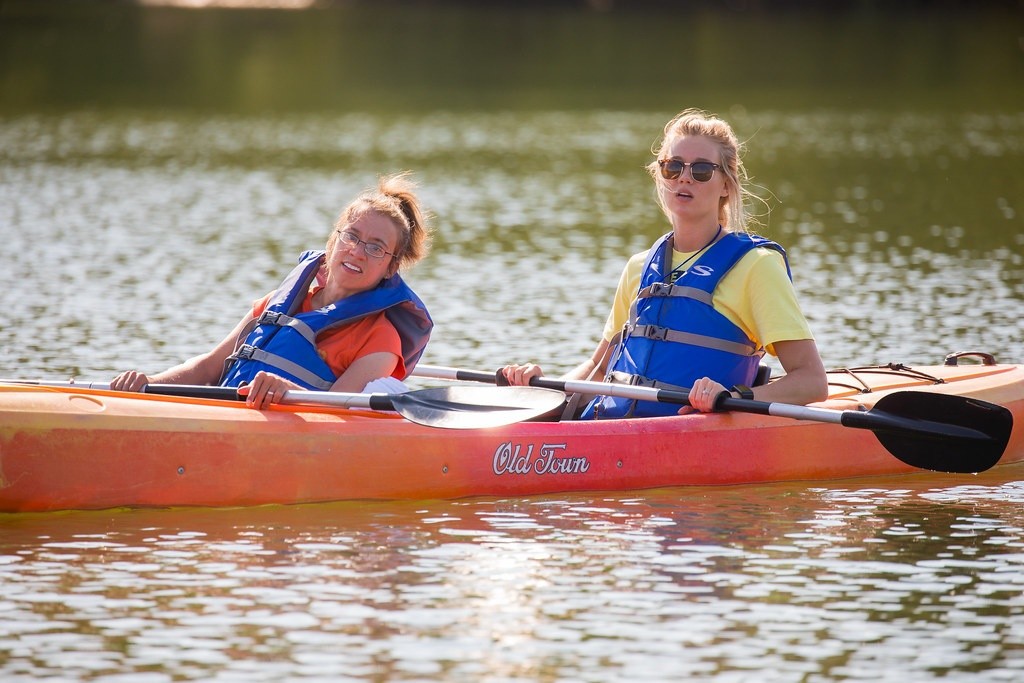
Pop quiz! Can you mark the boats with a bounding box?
[0,350,1024,513]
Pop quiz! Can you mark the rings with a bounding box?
[267,391,275,395]
[703,390,709,394]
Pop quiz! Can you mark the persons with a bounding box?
[110,171,433,410]
[502,107,828,420]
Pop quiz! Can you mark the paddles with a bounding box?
[410,363,1013,475]
[0,378,566,430]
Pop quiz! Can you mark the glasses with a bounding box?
[657,159,724,183]
[337,230,398,258]
